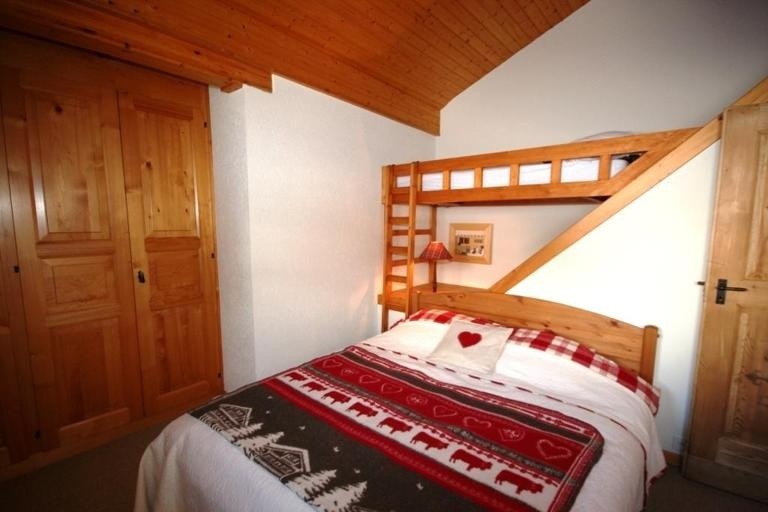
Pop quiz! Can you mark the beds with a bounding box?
[143,290,661,512]
[377,133,651,333]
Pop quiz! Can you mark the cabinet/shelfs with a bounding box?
[111,60,225,431]
[0,29,129,475]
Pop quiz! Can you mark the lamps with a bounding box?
[420,242,452,292]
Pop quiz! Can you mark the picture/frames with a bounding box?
[447,224,492,262]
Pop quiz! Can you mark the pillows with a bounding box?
[564,132,642,161]
[427,315,516,380]
